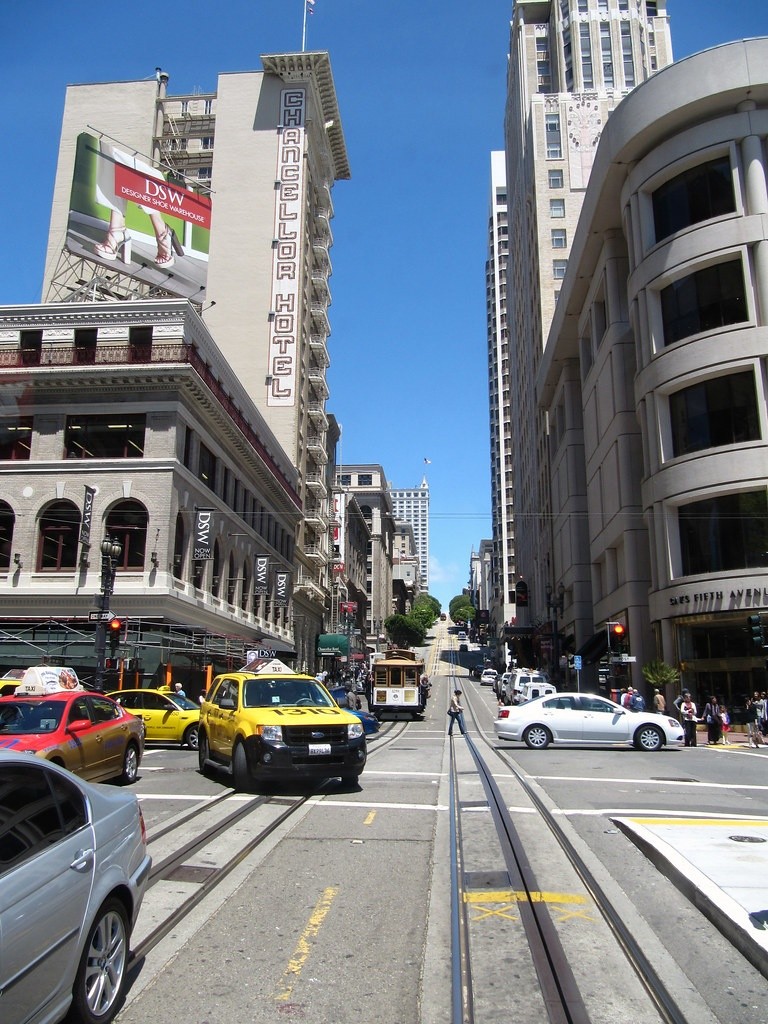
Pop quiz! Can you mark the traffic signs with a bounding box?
[88,610,116,623]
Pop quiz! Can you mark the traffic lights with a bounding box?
[105,658,119,669]
[110,619,120,648]
[124,658,139,671]
[614,625,625,651]
[748,614,764,646]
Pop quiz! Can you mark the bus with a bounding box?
[440,613,446,621]
[367,649,426,721]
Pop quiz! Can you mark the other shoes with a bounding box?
[714,743,718,745]
[706,742,711,745]
[756,746,759,748]
[722,741,731,745]
[748,745,752,748]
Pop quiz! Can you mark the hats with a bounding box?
[681,689,689,696]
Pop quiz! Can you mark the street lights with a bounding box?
[546,582,565,675]
[373,616,383,652]
[94,532,123,692]
[343,601,357,670]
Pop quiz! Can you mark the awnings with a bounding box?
[318,635,348,656]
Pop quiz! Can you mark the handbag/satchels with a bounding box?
[447,709,458,718]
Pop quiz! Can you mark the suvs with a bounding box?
[479,668,498,686]
[198,672,367,790]
[458,631,466,640]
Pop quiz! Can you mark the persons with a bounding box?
[420,671,431,687]
[199,689,206,705]
[469,664,473,676]
[624,687,633,708]
[621,688,628,706]
[493,661,507,674]
[674,688,689,726]
[680,693,697,747]
[508,663,512,672]
[653,689,666,714]
[701,696,732,745]
[343,662,370,711]
[174,683,185,709]
[316,668,343,686]
[630,689,645,712]
[448,690,469,736]
[93,141,184,267]
[741,692,768,748]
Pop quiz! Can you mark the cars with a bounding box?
[78,689,201,751]
[492,674,502,691]
[0,680,146,786]
[493,691,685,751]
[339,707,380,735]
[0,749,153,1024]
[474,665,485,675]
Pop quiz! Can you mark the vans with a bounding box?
[497,672,557,705]
